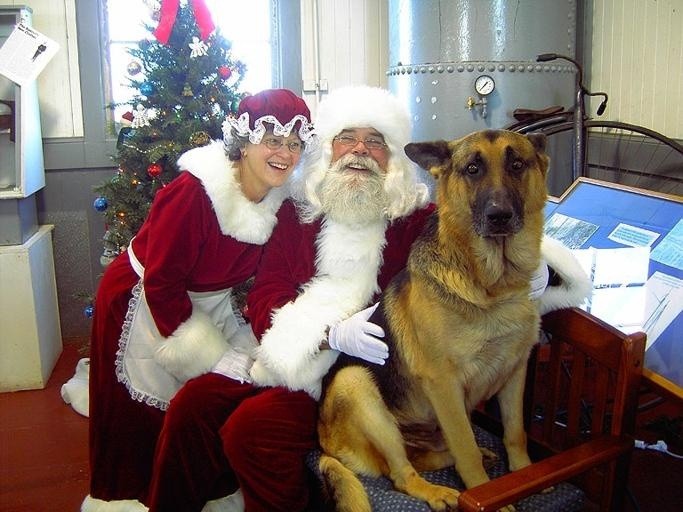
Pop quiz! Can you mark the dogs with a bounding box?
[318,130,551,512]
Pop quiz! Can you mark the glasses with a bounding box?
[261,138,304,154]
[334,135,388,149]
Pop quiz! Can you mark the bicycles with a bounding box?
[502,53,683,196]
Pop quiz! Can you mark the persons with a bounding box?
[147,82,593,512]
[80,89,315,511]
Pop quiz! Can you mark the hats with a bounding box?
[222,89,321,161]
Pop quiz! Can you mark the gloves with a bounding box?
[211,351,254,385]
[528,262,550,299]
[329,302,389,366]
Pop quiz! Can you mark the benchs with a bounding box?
[307,300,647,511]
